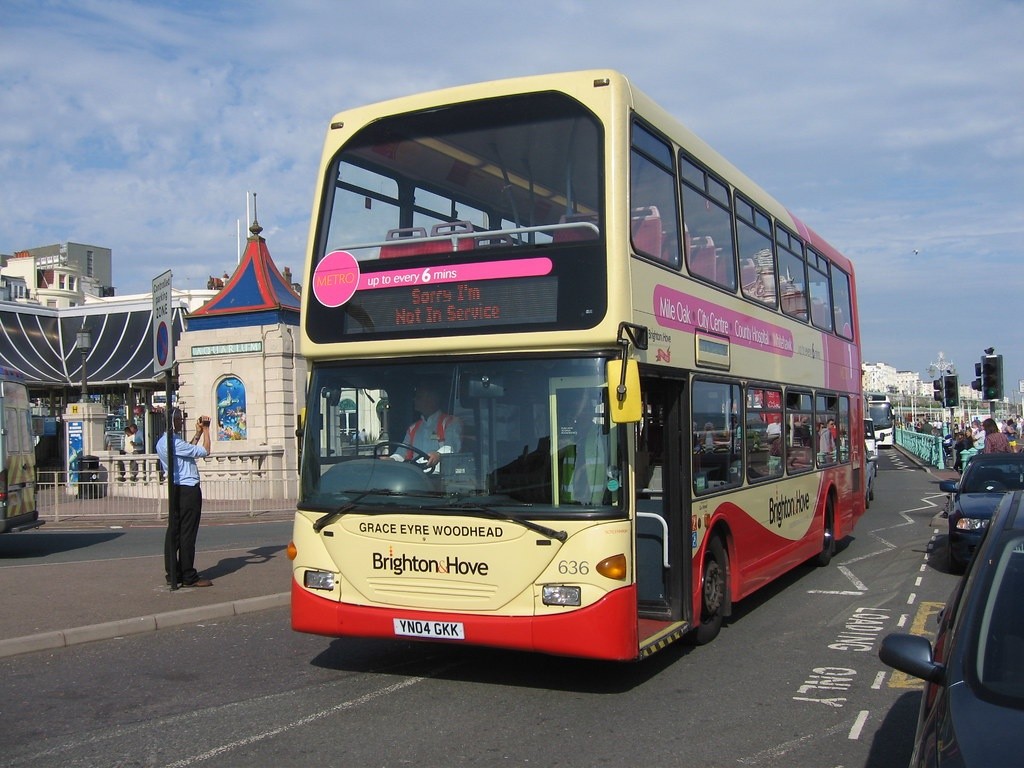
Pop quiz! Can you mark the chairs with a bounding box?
[458,424,481,457]
[381,206,852,342]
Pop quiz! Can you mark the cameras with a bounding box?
[199,417,202,423]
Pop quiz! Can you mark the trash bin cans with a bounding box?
[77,455,101,500]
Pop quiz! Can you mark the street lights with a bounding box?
[76,328,95,402]
[926,351,956,436]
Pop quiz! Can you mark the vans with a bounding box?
[0,380,46,533]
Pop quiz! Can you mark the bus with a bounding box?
[287,68,866,661]
[867,394,895,449]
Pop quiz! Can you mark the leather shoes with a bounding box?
[167,577,172,584]
[182,579,212,587]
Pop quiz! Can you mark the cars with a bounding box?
[939,452,1024,572]
[879,489,1024,768]
[864,418,884,477]
[865,442,879,509]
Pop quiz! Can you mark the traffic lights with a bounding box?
[972,363,981,391]
[944,375,959,408]
[933,376,945,408]
[981,355,1003,401]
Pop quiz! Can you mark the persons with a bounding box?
[130,424,144,479]
[124,427,134,456]
[359,429,368,443]
[378,383,463,475]
[692,412,845,474]
[896,414,1024,476]
[156,407,212,589]
[550,396,607,507]
